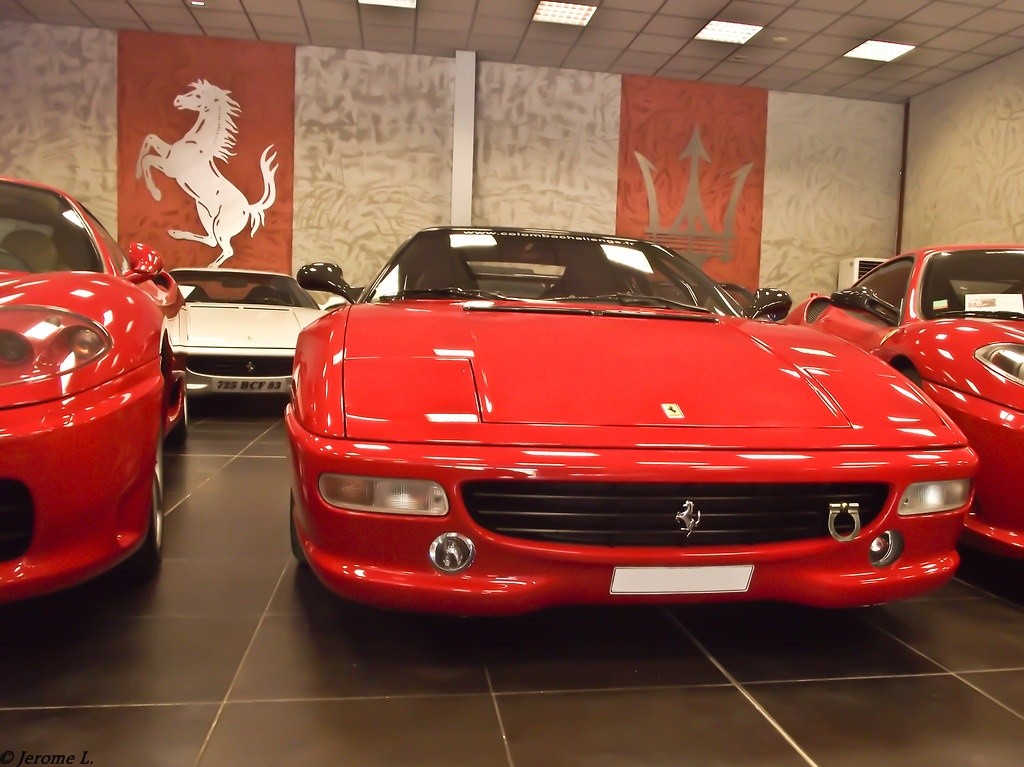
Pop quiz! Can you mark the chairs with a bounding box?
[180,282,288,306]
[423,249,645,298]
[5,227,61,274]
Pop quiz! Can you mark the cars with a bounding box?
[279,222,978,617]
[778,244,1024,562]
[161,266,326,412]
[0,176,190,604]
